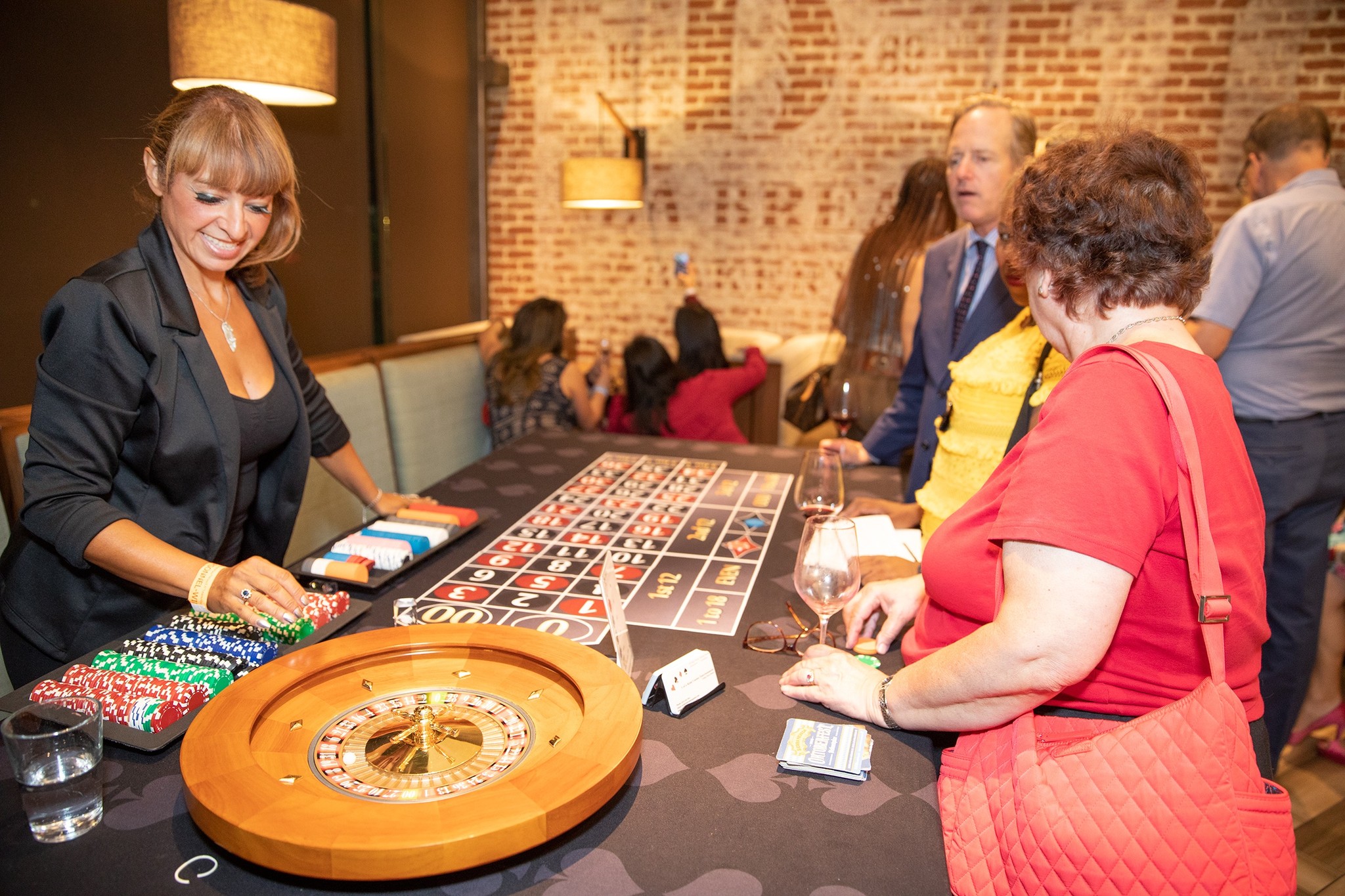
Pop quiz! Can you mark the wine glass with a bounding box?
[794,515,861,646]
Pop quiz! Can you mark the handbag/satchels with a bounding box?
[783,365,833,432]
[934,676,1296,896]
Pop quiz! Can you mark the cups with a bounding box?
[0,696,105,844]
[830,381,862,437]
[792,451,844,524]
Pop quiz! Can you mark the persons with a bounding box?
[483,298,614,453]
[820,156,957,444]
[831,161,1072,587]
[1,84,441,689]
[601,335,769,446]
[1182,103,1344,798]
[818,94,1038,504]
[777,123,1276,796]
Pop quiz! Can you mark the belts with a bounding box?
[1235,411,1345,425]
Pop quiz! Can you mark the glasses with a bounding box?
[743,599,836,658]
[1235,159,1252,197]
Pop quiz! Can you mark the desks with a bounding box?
[0,430,950,896]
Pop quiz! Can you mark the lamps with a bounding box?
[561,90,649,209]
[168,1,338,107]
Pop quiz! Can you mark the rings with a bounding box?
[240,588,257,604]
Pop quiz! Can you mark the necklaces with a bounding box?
[185,279,237,354]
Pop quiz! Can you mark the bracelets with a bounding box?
[187,562,228,614]
[879,675,904,730]
[362,488,382,524]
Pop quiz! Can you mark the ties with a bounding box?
[950,241,988,355]
